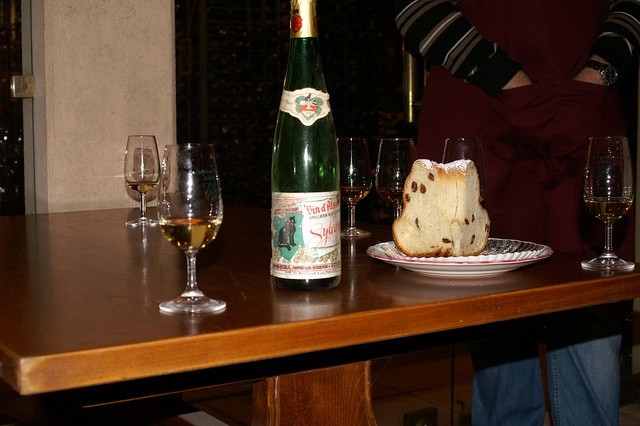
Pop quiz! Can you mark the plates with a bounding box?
[367,238,554,277]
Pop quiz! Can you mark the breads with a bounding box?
[392,158,492,256]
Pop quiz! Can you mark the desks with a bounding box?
[1,213,636,424]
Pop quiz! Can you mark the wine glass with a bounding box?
[158,144,226,315]
[581,134,638,272]
[375,137,421,221]
[123,134,161,228]
[337,138,373,238]
[440,138,487,199]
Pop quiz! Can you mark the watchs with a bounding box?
[584,59,619,86]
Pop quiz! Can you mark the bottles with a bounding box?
[271,1,343,290]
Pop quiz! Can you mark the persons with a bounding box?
[390,0,639,426]
